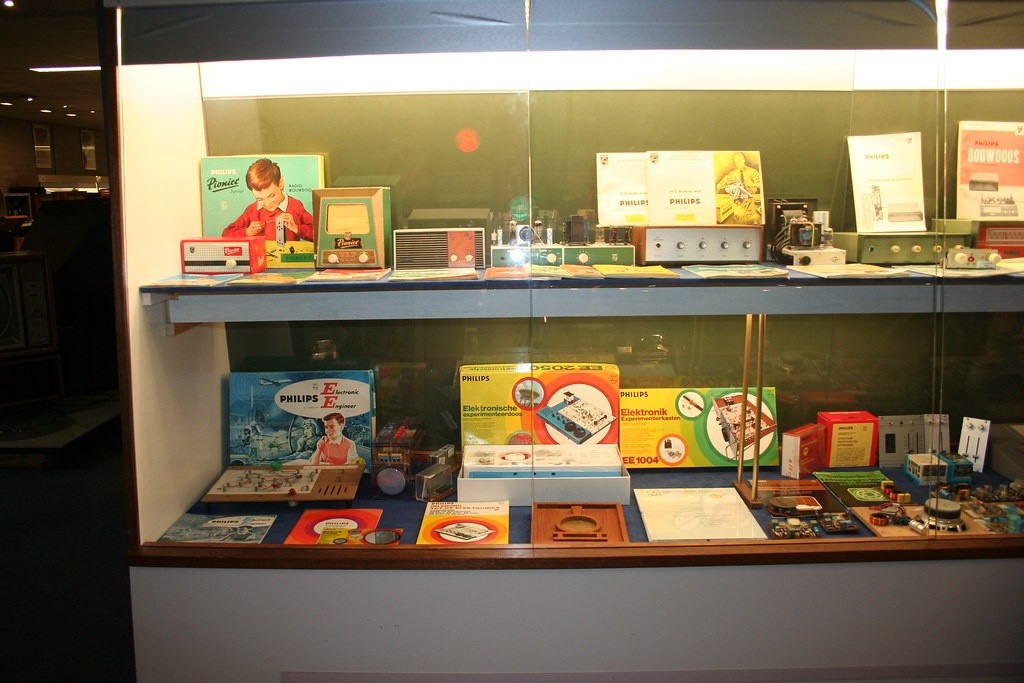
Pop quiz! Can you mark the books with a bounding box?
[226,267,478,282]
[633,487,768,541]
[683,263,791,280]
[416,501,509,545]
[786,262,911,280]
[596,150,766,229]
[200,153,326,269]
[157,512,277,545]
[891,254,1024,279]
[142,273,243,288]
[748,469,924,518]
[955,120,1024,222]
[283,507,383,544]
[483,263,681,283]
[846,131,928,233]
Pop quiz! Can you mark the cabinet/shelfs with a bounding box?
[110,0,1024,683]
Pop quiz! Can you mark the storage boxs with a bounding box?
[229,370,377,475]
[816,410,878,468]
[200,153,325,267]
[617,388,780,468]
[311,187,394,270]
[934,450,972,485]
[904,451,946,485]
[457,446,631,507]
[459,362,621,449]
[780,422,826,479]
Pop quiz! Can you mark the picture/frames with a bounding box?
[80,128,97,171]
[32,122,52,170]
